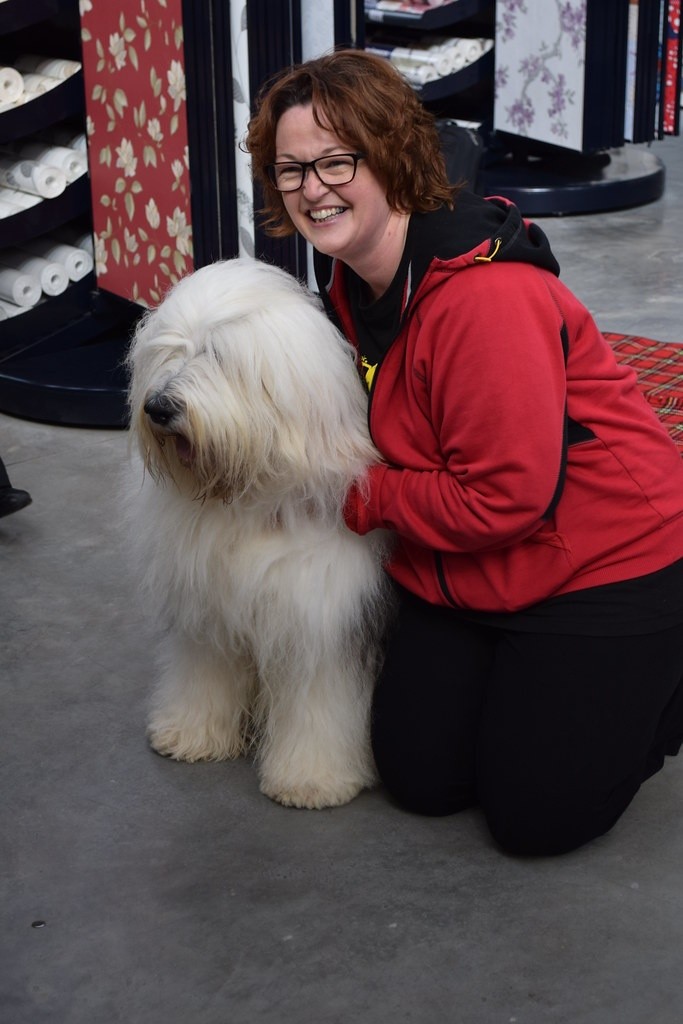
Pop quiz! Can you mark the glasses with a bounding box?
[263,152,366,193]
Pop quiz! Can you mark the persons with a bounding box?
[244,51,683,858]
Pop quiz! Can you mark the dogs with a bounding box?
[120,259,397,812]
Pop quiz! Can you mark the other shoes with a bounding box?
[0,487,31,518]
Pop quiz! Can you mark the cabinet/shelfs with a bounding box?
[0,0,665,429]
